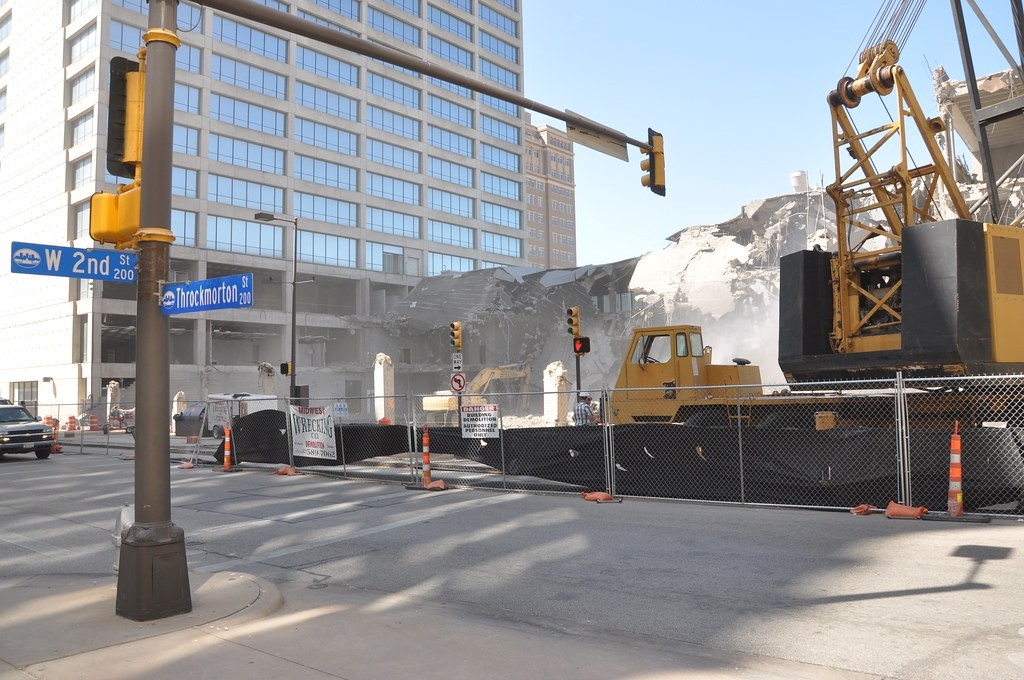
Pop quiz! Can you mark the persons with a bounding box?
[573,392,601,427]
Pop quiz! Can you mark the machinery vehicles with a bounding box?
[606,1,1024,428]
[422,363,528,426]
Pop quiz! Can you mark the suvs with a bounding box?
[0,404,54,460]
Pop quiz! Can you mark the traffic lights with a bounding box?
[449,321,461,351]
[566,306,580,336]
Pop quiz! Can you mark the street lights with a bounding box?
[255,212,299,405]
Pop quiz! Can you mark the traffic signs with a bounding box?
[452,353,462,372]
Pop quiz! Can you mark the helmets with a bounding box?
[579,392,590,397]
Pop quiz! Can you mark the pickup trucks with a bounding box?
[109,405,135,434]
